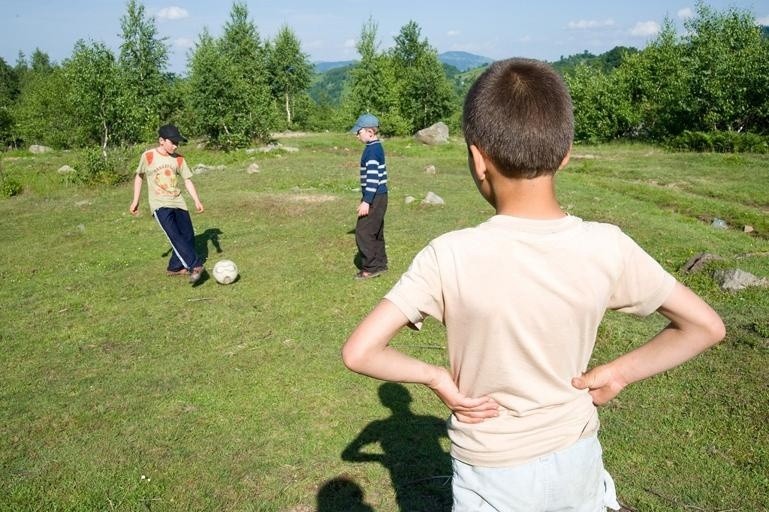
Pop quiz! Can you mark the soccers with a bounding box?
[214,260,236,284]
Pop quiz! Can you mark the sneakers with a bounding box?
[353,263,389,281]
[167,267,191,276]
[188,262,204,286]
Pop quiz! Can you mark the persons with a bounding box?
[343,58,728,511]
[130,125,211,283]
[350,114,388,279]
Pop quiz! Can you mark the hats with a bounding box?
[348,113,380,136]
[157,124,188,146]
